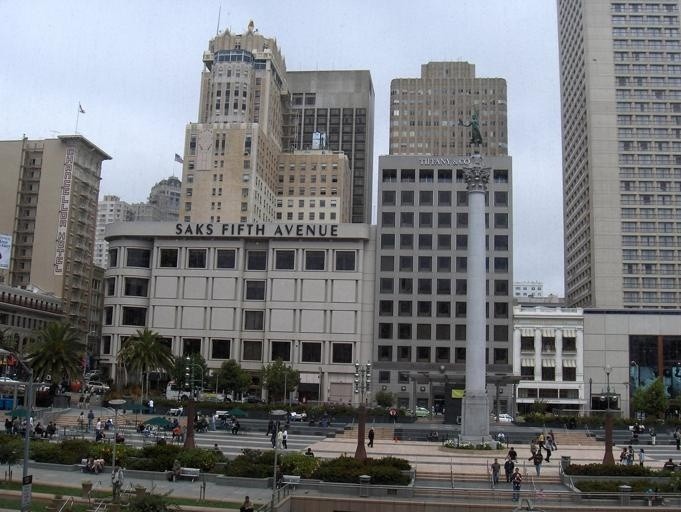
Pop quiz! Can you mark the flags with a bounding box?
[79,102,86,114]
[175,154,184,165]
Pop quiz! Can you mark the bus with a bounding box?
[166,382,233,402]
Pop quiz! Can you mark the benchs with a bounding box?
[77,459,104,473]
[665,464,681,471]
[629,426,636,431]
[179,466,200,482]
[281,475,301,491]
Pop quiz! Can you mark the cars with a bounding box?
[85,382,110,393]
[409,407,431,416]
[493,413,514,423]
[81,377,94,382]
[273,399,303,405]
[0,376,19,383]
[87,370,103,377]
[244,397,266,404]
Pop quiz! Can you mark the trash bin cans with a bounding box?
[619,485,632,506]
[360,475,371,497]
[561,456,570,472]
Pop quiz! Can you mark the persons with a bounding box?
[241,495,255,512]
[461,111,481,144]
[77,410,111,441]
[172,426,184,442]
[214,443,219,450]
[109,462,125,504]
[177,405,184,415]
[5,416,57,439]
[489,422,681,506]
[290,410,335,426]
[87,455,103,474]
[195,414,208,432]
[305,448,314,459]
[137,421,144,432]
[148,398,155,413]
[266,407,290,450]
[224,415,240,435]
[78,393,90,409]
[275,465,284,489]
[169,460,180,481]
[116,432,126,442]
[367,426,376,448]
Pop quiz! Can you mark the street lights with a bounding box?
[282,370,289,406]
[107,397,126,502]
[602,365,620,464]
[82,329,96,374]
[631,360,642,429]
[184,351,205,450]
[0,347,33,512]
[353,360,373,461]
[268,408,288,505]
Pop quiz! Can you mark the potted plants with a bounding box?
[82,480,93,493]
[134,483,146,497]
